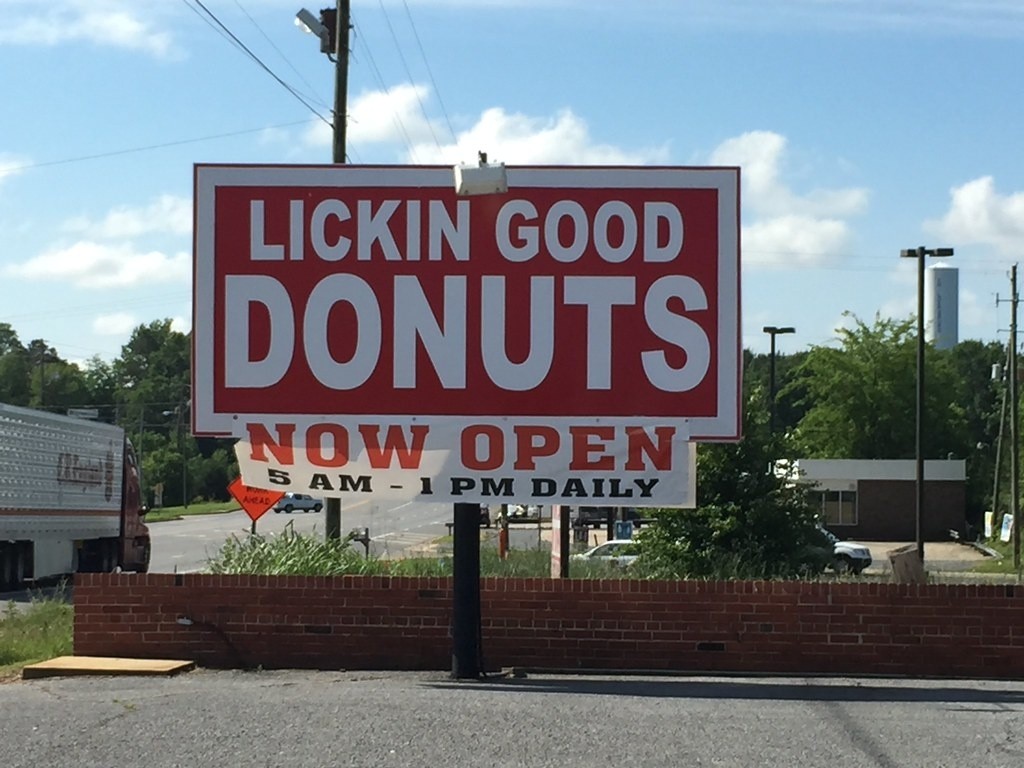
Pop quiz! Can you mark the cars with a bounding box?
[573,537,645,569]
[820,541,875,580]
[273,487,322,513]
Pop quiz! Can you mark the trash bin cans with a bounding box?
[888,545,923,582]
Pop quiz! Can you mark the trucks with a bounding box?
[2,401,151,597]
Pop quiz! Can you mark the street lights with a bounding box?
[899,246,956,563]
[765,326,795,484]
